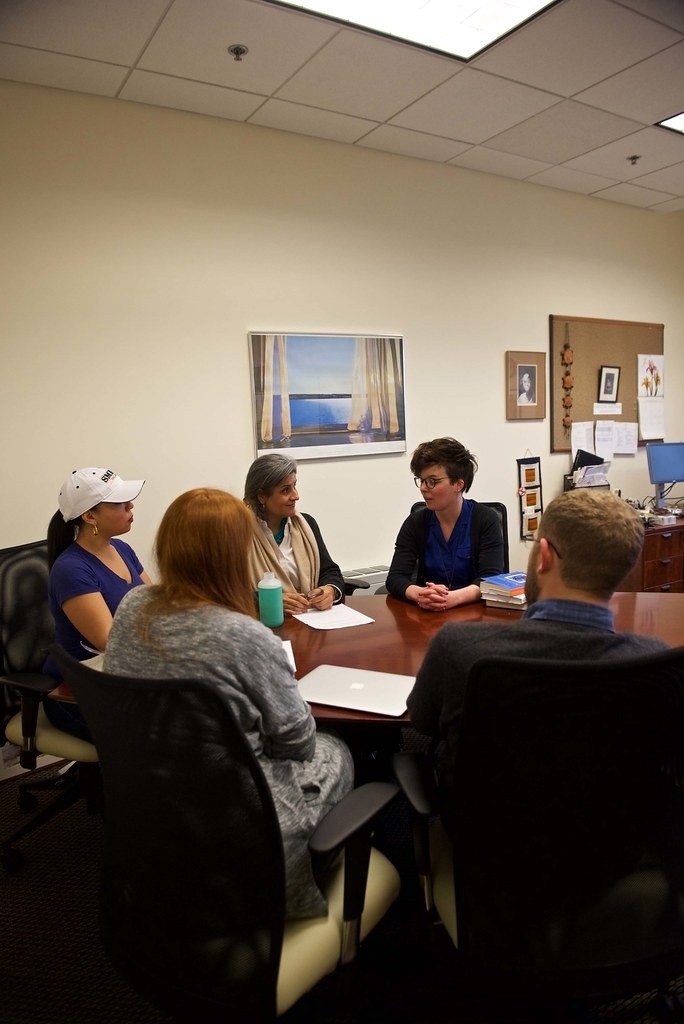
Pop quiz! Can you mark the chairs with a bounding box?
[47,643,402,1024]
[388,647,684,1024]
[0,537,61,768]
[374,502,509,595]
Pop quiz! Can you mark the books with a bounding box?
[480,572,528,610]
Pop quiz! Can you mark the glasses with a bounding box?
[523,532,564,560]
[413,476,449,490]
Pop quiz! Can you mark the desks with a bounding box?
[266,593,684,785]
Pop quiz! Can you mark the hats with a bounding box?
[57,468,146,523]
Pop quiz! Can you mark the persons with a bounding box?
[244,453,347,613]
[47,468,149,697]
[518,369,536,403]
[407,491,655,783]
[103,489,356,918]
[386,437,503,611]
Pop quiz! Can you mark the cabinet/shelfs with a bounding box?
[612,515,684,594]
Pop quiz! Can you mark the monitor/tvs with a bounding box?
[646,442,684,508]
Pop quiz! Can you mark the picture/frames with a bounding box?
[598,365,620,403]
[246,331,408,461]
[506,350,546,420]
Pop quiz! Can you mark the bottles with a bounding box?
[258,572,284,627]
